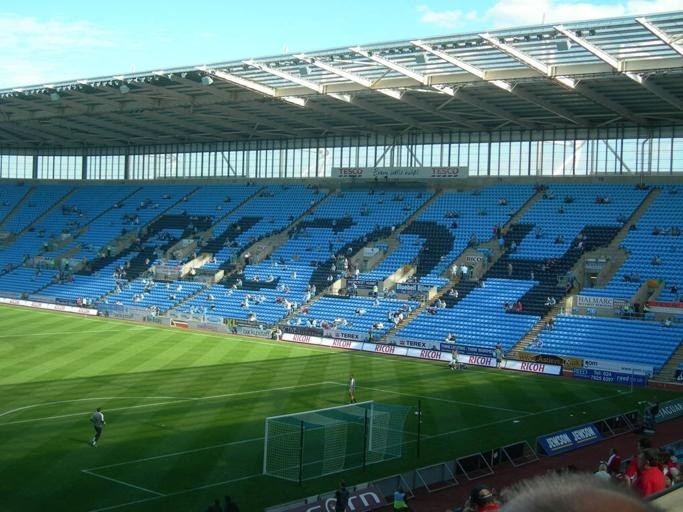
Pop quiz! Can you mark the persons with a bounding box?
[394,486,408,509]
[208,495,238,512]
[464,439,682,512]
[335,480,349,511]
[89,408,105,447]
[348,375,358,404]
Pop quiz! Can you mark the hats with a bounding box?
[470,484,492,503]
